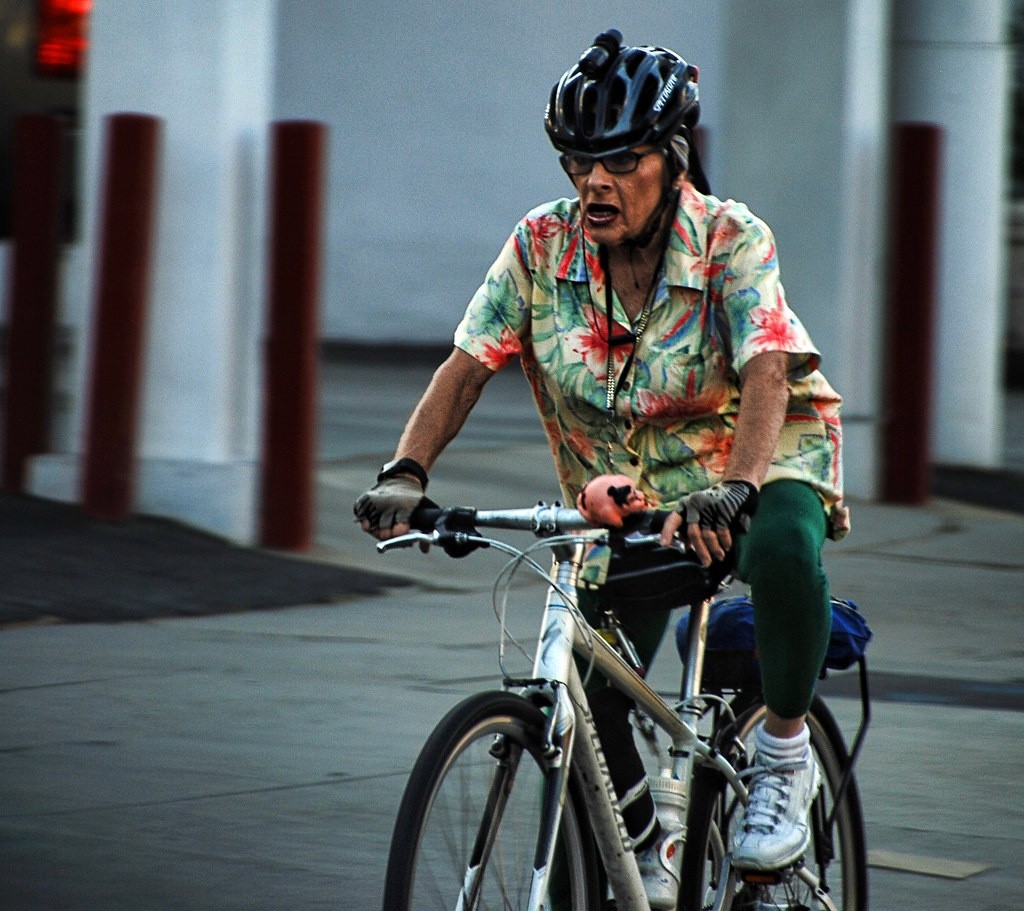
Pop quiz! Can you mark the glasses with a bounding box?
[559,147,670,176]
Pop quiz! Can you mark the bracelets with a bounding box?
[376,458,429,491]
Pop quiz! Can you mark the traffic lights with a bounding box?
[1,0,93,240]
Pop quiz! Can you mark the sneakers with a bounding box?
[606,822,744,910]
[724,718,823,872]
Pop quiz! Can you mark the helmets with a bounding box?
[543,28,700,158]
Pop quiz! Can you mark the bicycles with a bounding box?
[352,506,874,911]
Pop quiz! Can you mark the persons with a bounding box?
[355,26,845,911]
